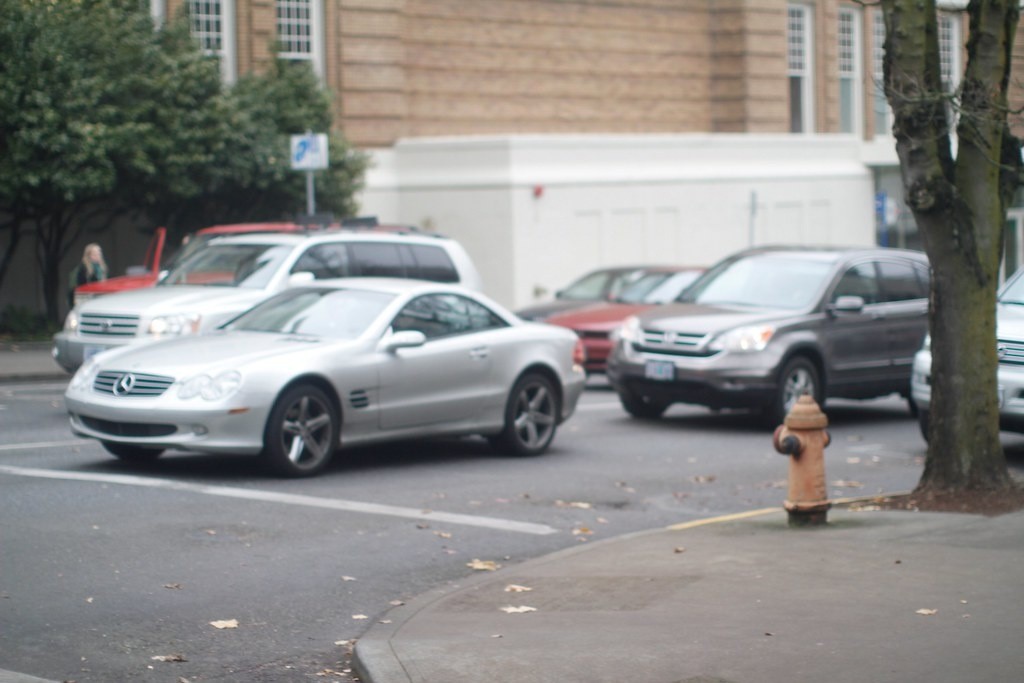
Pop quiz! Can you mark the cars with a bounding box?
[507,264,728,375]
[909,267,1024,447]
[613,247,941,428]
[60,267,588,481]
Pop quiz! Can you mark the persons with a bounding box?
[67,243,107,310]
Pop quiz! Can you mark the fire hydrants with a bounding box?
[772,389,834,527]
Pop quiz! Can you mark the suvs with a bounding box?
[72,217,418,326]
[52,229,480,383]
